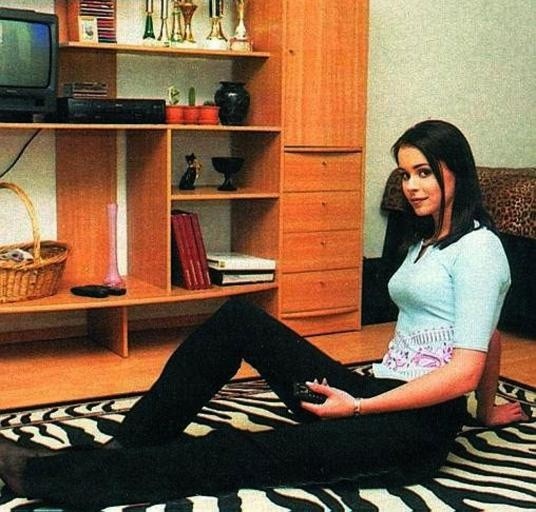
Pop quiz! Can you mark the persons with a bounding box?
[1,118,528,503]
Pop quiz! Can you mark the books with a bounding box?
[64,1,118,47]
[164,205,280,291]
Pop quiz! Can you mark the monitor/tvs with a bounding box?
[0,9,60,122]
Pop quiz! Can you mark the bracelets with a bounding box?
[352,395,366,419]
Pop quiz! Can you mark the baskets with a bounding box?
[0,181,68,304]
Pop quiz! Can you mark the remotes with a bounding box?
[292,382,327,403]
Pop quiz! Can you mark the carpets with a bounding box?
[0,356,536,512]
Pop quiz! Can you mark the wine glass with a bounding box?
[212,157,245,192]
